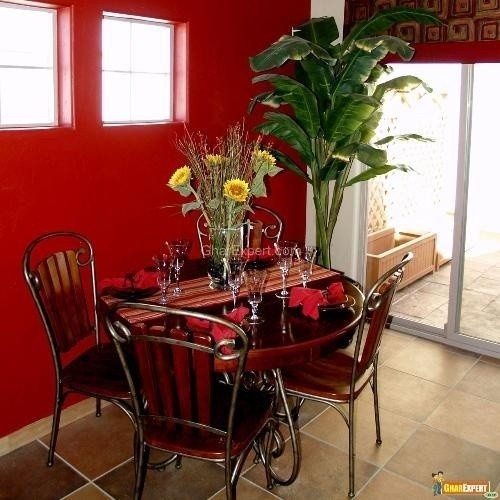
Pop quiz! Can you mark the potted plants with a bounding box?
[247,6,449,362]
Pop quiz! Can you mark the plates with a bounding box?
[318,295,356,313]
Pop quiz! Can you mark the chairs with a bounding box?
[23,229,413,499]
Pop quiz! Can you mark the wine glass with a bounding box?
[152,239,318,325]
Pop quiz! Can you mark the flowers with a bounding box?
[168,147,282,284]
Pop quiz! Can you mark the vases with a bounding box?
[206,226,245,291]
